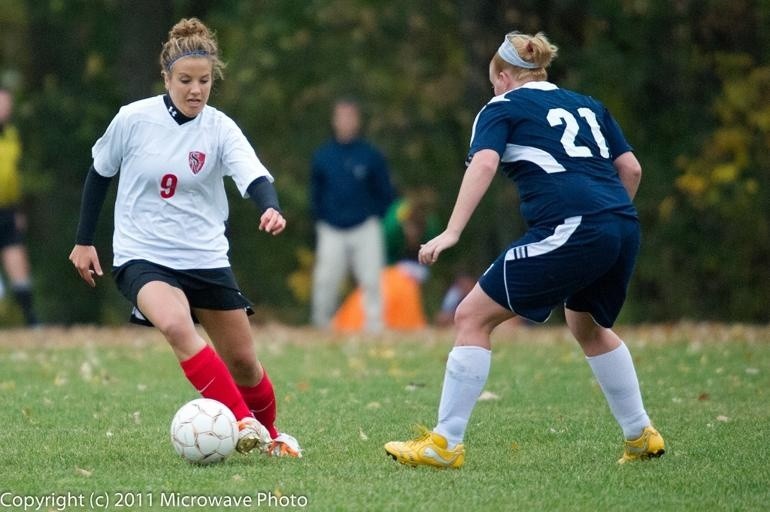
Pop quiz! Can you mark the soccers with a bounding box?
[170,398,239,463]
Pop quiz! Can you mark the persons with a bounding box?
[0,87,36,329]
[382,30,666,468]
[329,250,432,329]
[308,92,398,332]
[69,16,302,461]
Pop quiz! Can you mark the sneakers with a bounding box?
[235,417,302,458]
[617,424,666,464]
[384,434,466,469]
